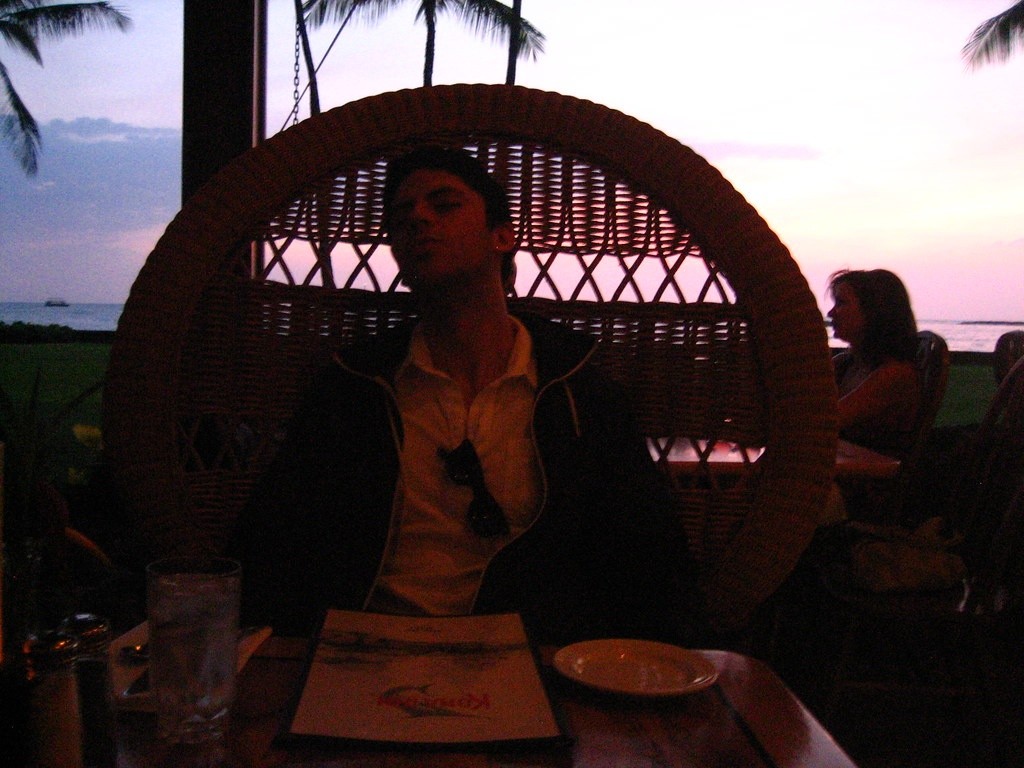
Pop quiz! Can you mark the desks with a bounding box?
[644,435,902,479]
[104,630,859,768]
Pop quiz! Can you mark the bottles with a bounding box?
[17,632,87,768]
[55,613,117,768]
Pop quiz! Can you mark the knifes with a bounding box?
[117,669,157,705]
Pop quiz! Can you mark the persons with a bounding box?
[223,145,706,647]
[816,271,920,532]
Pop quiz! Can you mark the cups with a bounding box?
[142,553,241,745]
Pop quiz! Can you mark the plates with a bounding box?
[553,638,719,696]
[288,609,560,745]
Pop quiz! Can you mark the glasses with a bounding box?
[443,438,513,537]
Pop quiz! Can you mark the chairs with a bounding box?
[103,84,839,645]
[770,330,953,648]
[792,352,1024,768]
